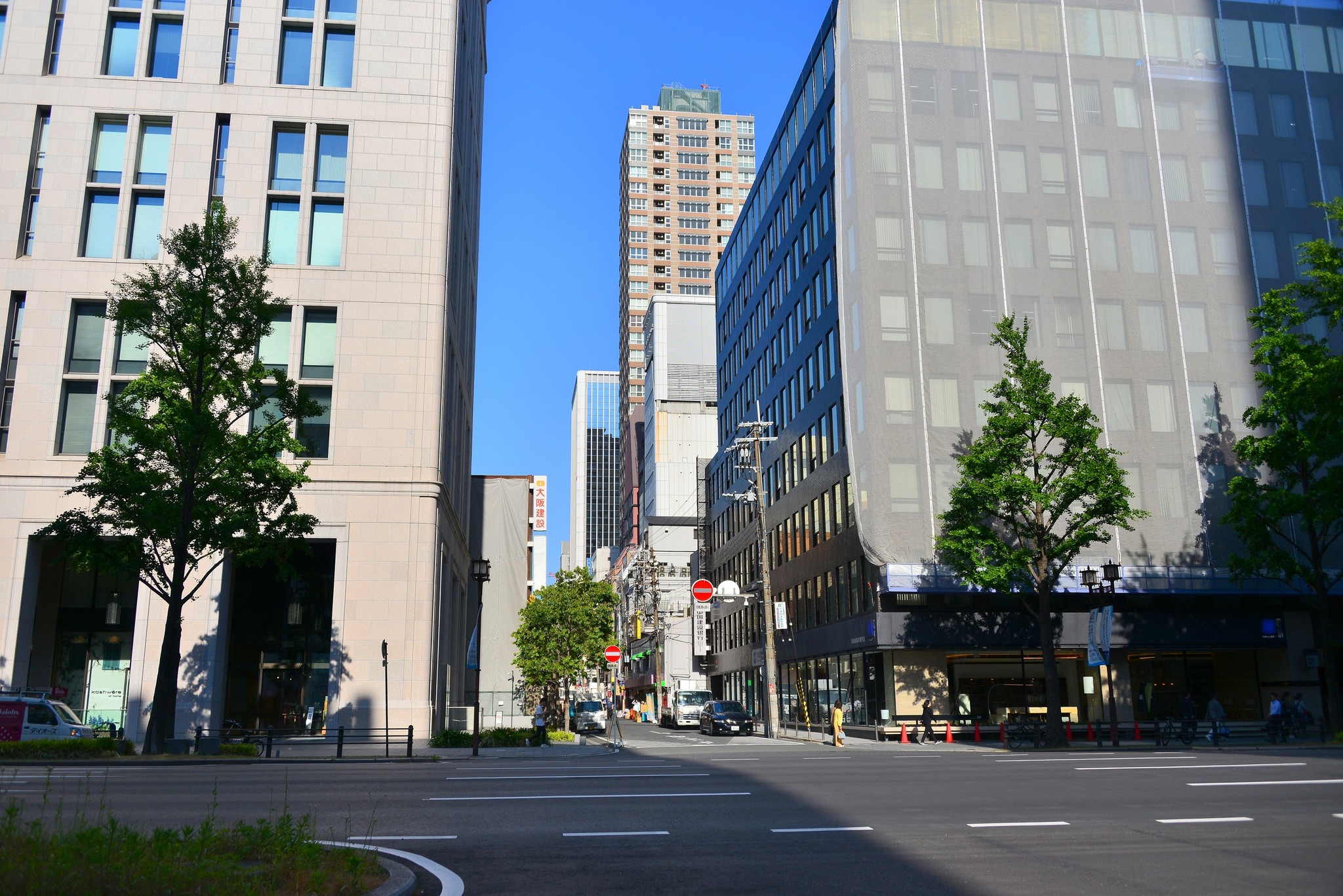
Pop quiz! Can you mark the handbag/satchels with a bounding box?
[838,729,846,739]
[535,710,545,719]
[828,725,834,736]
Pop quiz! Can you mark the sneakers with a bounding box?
[838,745,844,747]
[1205,735,1212,743]
[526,739,530,747]
[842,744,845,746]
[920,741,928,745]
[541,744,549,748]
[935,740,942,745]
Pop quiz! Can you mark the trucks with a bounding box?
[659,689,713,730]
[789,686,853,724]
[573,691,607,735]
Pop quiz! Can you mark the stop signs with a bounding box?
[692,580,713,602]
[604,645,621,663]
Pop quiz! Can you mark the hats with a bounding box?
[641,701,645,704]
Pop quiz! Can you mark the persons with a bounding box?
[1205,693,1228,742]
[1182,693,1195,719]
[791,697,797,706]
[633,704,641,722]
[1265,691,1309,746]
[632,699,640,705]
[606,699,614,720]
[919,700,942,745]
[834,701,845,747]
[602,699,607,719]
[641,701,645,705]
[831,699,844,744]
[526,698,551,748]
[854,697,862,725]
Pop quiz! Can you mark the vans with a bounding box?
[0,686,93,741]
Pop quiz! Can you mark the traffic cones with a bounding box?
[1133,721,1142,741]
[1064,721,1075,740]
[1085,721,1095,741]
[970,722,986,743]
[897,723,913,744]
[996,723,1007,742]
[945,723,956,743]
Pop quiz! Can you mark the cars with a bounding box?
[699,701,755,737]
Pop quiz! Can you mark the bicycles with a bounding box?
[1008,714,1059,750]
[224,718,266,758]
[1159,715,1198,746]
[95,722,121,739]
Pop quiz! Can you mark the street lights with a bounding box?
[1080,561,1124,746]
[471,554,492,757]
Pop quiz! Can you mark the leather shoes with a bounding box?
[1264,734,1309,746]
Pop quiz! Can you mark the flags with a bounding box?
[466,604,483,669]
[575,684,587,687]
